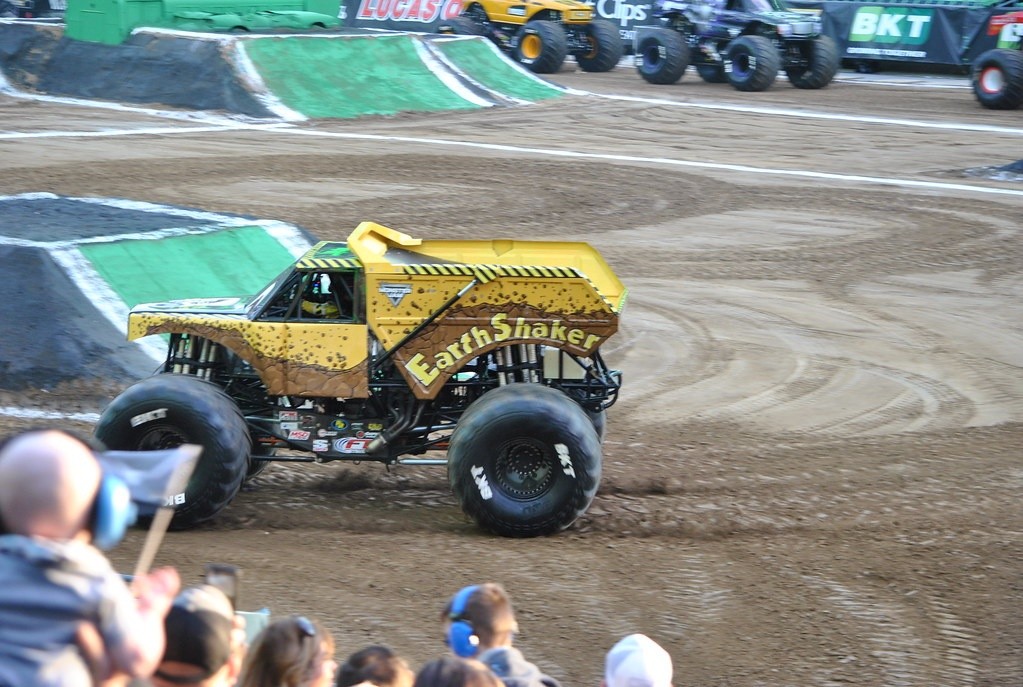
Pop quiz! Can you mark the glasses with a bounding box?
[294,616,316,647]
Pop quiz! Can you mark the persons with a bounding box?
[0,429,674,687]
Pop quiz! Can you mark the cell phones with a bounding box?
[205,565,238,610]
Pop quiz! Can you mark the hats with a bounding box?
[163,584,235,672]
[603,633,673,687]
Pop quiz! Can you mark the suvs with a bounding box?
[632,1,838,93]
[94,221,630,541]
[435,1,624,74]
[967,1,1022,110]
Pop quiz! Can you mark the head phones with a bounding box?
[447,585,479,658]
[0,428,137,551]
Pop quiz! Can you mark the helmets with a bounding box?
[301,273,337,316]
